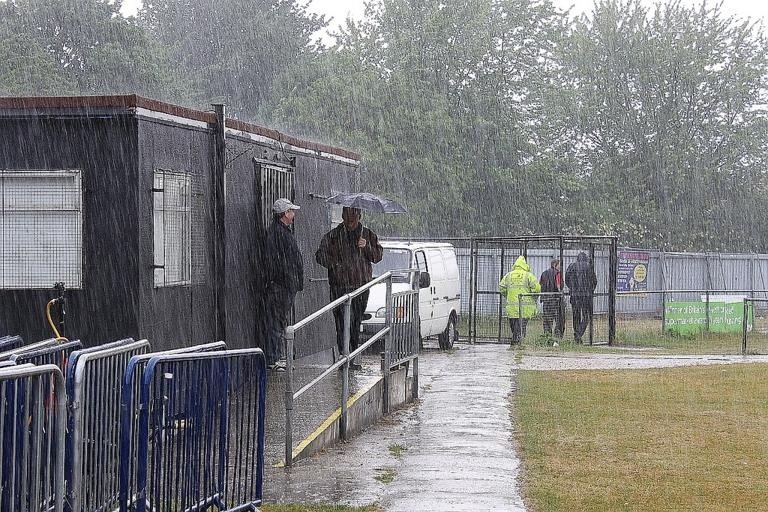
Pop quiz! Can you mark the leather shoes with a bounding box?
[338,358,363,371]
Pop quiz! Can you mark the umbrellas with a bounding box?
[327,191,408,258]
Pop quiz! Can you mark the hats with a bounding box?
[271,198,300,214]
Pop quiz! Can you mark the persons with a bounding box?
[499,255,541,350]
[255,198,303,372]
[539,259,567,348]
[316,206,383,370]
[565,252,597,346]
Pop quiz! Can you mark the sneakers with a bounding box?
[262,357,296,372]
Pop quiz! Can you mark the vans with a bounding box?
[357,241,461,351]
